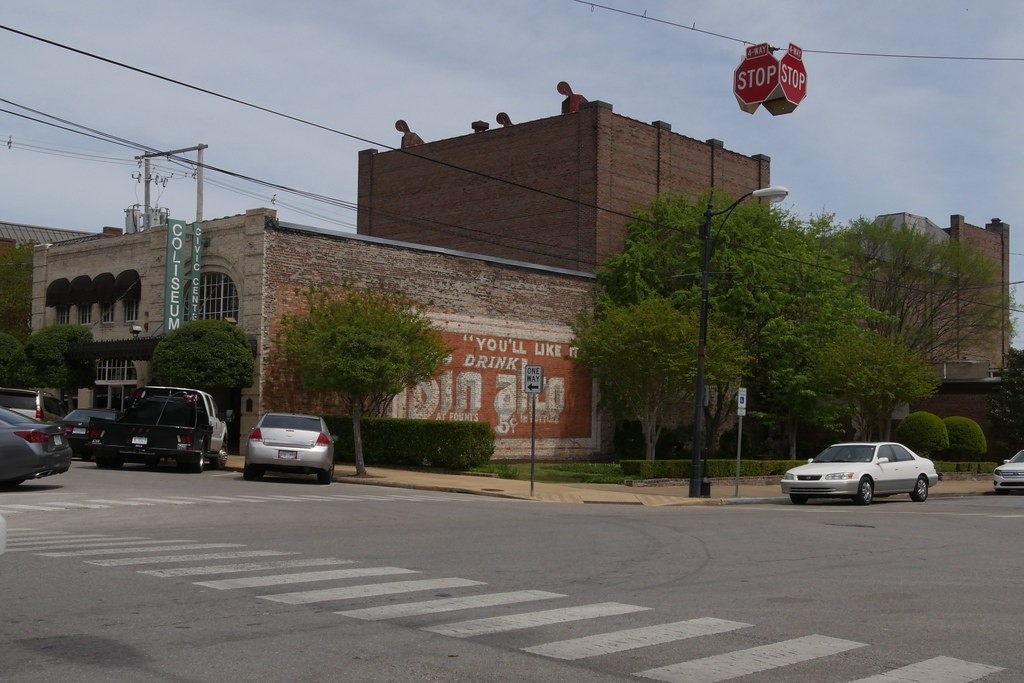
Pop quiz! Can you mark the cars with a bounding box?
[243,412,339,485]
[0,405,72,482]
[781,442,938,506]
[993,450,1024,494]
[62,408,123,460]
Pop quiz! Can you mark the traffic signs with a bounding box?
[525,365,542,393]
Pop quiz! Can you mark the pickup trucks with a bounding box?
[91,386,228,473]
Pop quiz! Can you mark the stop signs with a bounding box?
[779,43,808,106]
[733,43,780,105]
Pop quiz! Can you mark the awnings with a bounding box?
[45,269,140,308]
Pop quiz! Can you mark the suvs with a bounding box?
[0,387,68,424]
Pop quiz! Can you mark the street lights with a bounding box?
[688,185,789,499]
